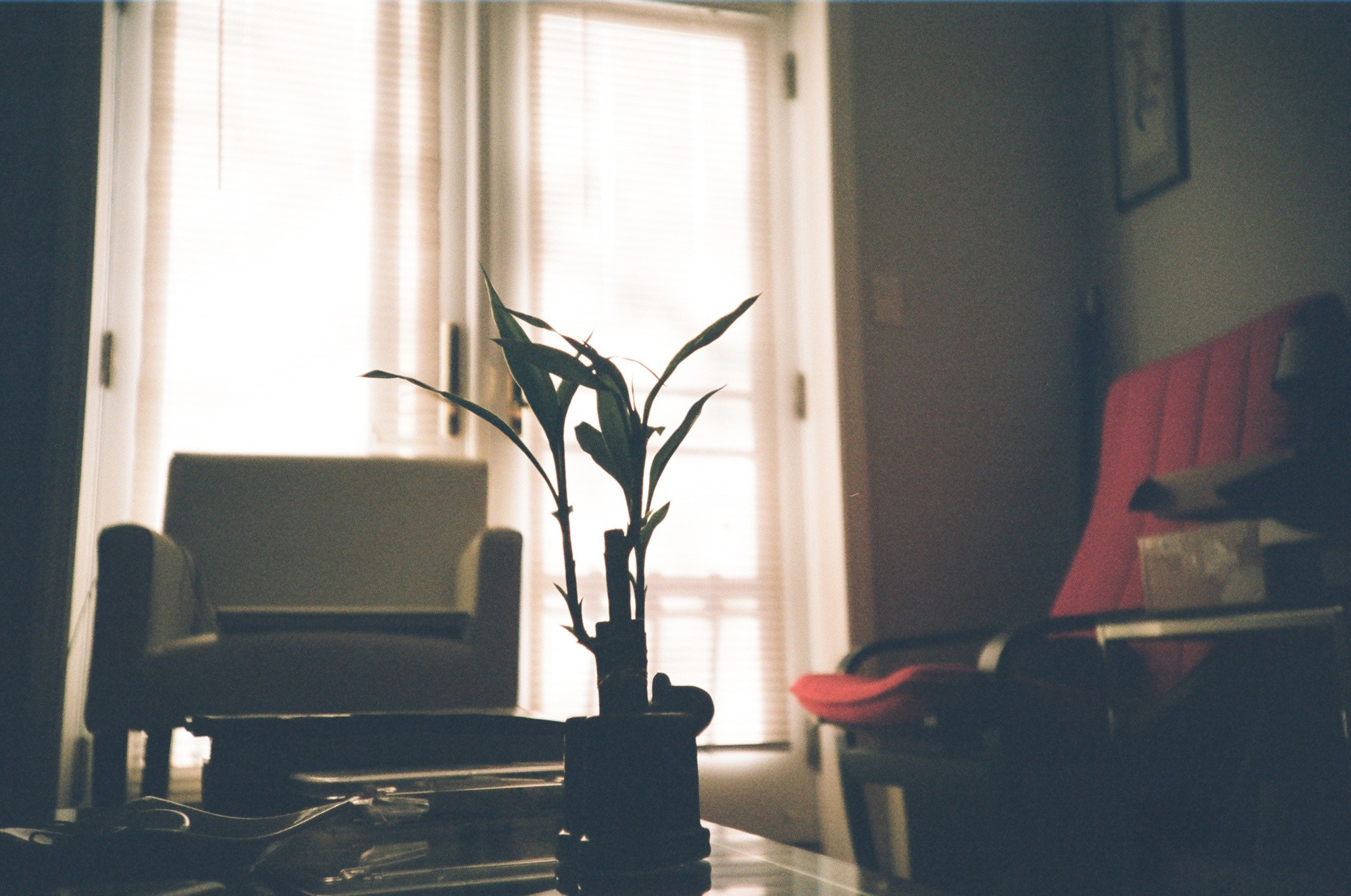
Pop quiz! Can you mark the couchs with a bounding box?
[82,453,522,808]
[790,297,1351,896]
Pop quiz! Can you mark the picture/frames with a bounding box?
[1102,0,1188,213]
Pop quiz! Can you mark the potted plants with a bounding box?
[365,260,760,894]
